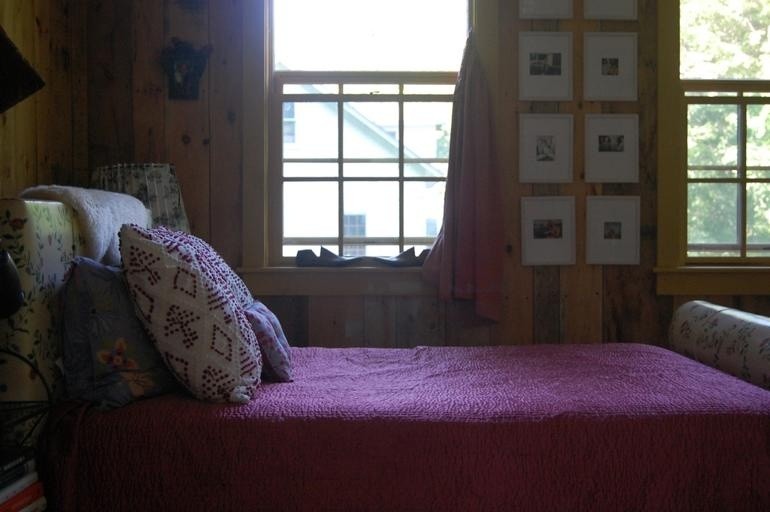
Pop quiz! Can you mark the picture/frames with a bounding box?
[518,0,574,20]
[518,31,573,102]
[518,111,574,183]
[585,195,641,265]
[583,31,638,103]
[520,196,576,266]
[584,113,639,183]
[583,0,638,21]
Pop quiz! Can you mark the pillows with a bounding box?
[244,297,294,385]
[72,256,164,405]
[119,222,263,405]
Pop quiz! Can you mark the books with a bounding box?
[0,443,48,512]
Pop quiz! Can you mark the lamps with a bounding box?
[91,162,192,234]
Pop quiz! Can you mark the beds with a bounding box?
[0,186,769,512]
